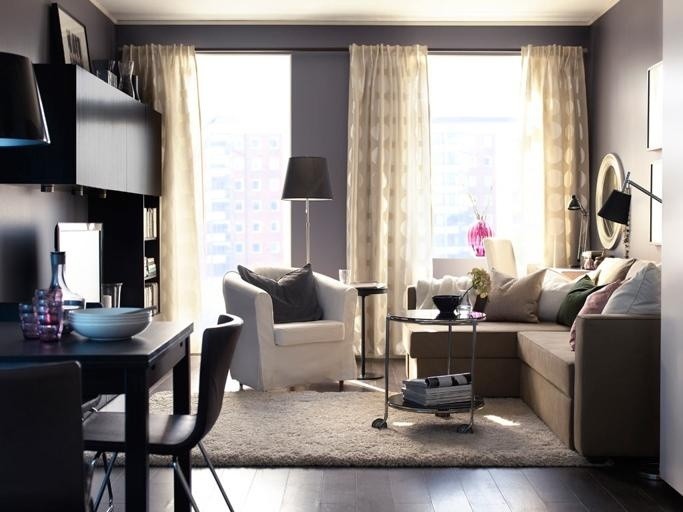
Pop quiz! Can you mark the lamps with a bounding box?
[567,195,588,259]
[281,157,334,270]
[0,51,51,148]
[596,172,662,225]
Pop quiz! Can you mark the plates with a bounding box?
[67,306,151,342]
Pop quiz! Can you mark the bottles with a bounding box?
[33,250,86,336]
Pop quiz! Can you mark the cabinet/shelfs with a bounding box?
[89,195,161,316]
[0,65,161,197]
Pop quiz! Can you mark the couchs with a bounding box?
[403,259,659,464]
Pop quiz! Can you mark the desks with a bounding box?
[356,284,386,382]
[0,320,194,512]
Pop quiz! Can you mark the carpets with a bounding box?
[80,390,608,469]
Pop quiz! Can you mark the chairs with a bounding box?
[0,361,92,512]
[222,266,355,392]
[482,238,517,278]
[80,312,245,512]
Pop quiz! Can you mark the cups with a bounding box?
[338,268,352,286]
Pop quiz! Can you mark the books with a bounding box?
[143,208,158,317]
[400,373,476,405]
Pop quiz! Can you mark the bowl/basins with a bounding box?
[432,295,463,313]
[18,302,42,340]
[33,287,63,342]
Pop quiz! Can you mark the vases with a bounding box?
[456,289,473,320]
[117,61,135,97]
[467,218,496,258]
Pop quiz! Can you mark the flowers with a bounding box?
[458,267,492,302]
[465,184,496,223]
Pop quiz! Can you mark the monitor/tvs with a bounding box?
[53,222,104,309]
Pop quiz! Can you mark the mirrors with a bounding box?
[594,153,626,250]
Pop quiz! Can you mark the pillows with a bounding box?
[480,262,660,353]
[237,265,325,322]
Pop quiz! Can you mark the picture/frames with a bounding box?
[648,159,664,245]
[52,1,91,74]
[644,60,663,151]
